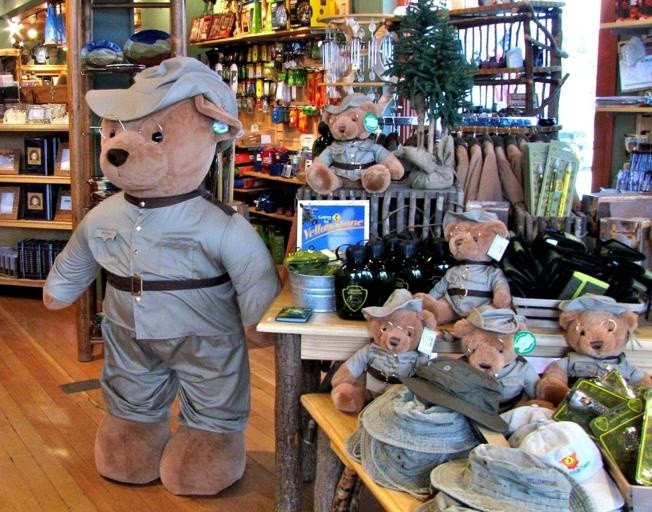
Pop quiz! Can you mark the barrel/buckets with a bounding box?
[290,269,337,313]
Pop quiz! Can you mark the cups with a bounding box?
[27,104,66,123]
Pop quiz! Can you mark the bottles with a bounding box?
[254,149,262,171]
[332,230,458,322]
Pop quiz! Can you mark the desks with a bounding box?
[298,388,439,511]
[255,269,652,512]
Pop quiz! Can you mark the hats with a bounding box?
[343,358,508,494]
[415,406,625,511]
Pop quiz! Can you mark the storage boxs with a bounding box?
[596,442,652,512]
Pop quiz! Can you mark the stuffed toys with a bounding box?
[535,291,652,405]
[412,208,512,325]
[451,304,540,416]
[307,92,406,196]
[42,57,282,497]
[331,289,437,415]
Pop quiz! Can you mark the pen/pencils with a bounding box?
[538,159,572,217]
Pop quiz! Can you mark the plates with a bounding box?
[80,39,124,69]
[124,29,171,67]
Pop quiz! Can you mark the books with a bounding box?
[275,307,312,323]
[21,183,52,221]
[47,137,56,176]
[23,138,47,176]
[0,238,69,280]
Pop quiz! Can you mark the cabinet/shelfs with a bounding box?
[189,1,570,287]
[60,0,190,363]
[0,1,77,295]
[590,0,652,194]
